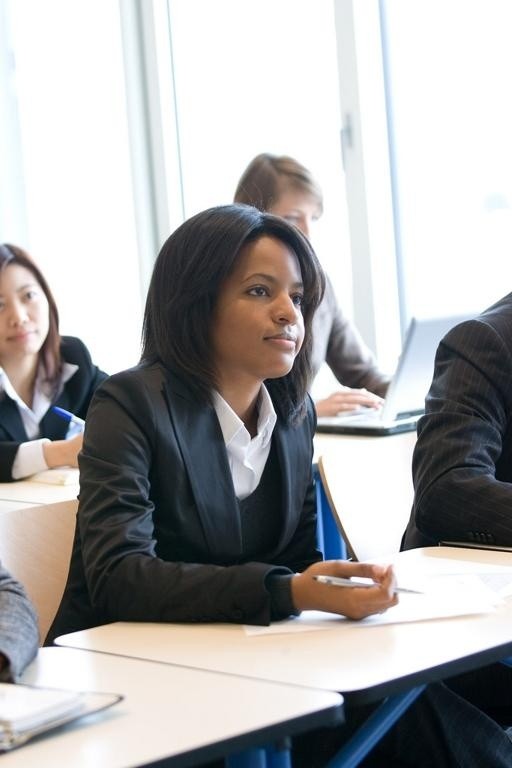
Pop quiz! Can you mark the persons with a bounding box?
[0,245,110,484]
[234,155,391,417]
[399,291,511,728]
[0,573,39,682]
[43,203,512,768]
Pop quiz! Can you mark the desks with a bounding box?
[53,545,512,706]
[0,645,346,767]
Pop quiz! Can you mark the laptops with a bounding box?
[315,312,476,438]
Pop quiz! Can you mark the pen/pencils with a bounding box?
[312,575,428,596]
[51,405,85,428]
